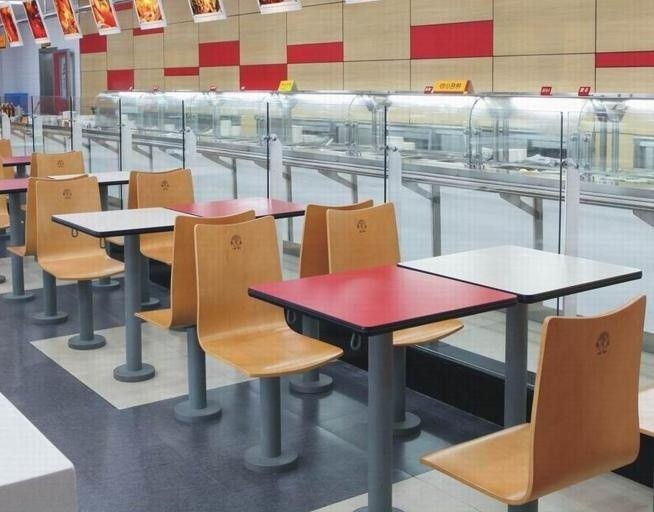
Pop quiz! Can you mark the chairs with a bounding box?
[2,136,375,424]
[326,203,464,437]
[193,215,343,475]
[420,293,646,511]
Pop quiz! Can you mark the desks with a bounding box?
[248,264,518,512]
[397,244,643,428]
